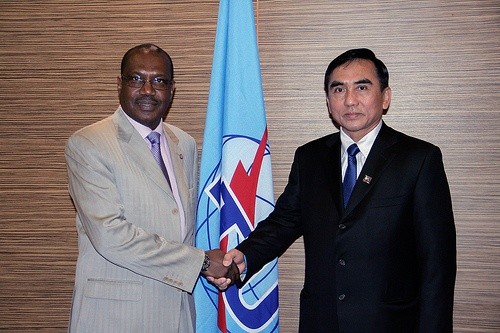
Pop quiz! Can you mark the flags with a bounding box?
[193,1,280,333]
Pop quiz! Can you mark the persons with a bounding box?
[205,48,458,333]
[63,43,242,333]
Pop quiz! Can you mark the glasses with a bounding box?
[121,75,172,90]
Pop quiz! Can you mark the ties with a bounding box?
[146,132,173,193]
[342,143,360,208]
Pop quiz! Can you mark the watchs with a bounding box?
[201,250,211,272]
